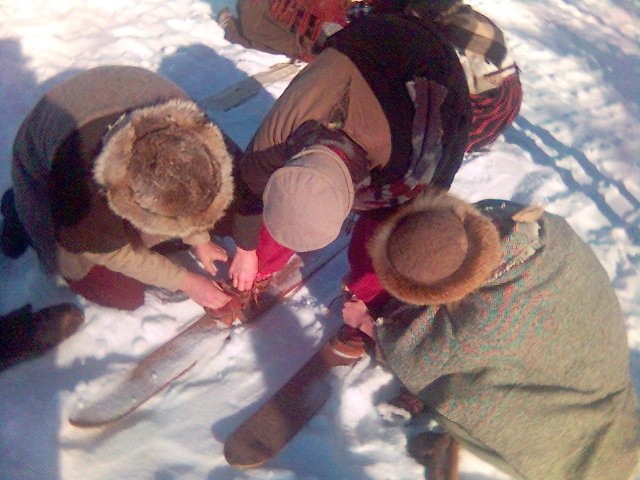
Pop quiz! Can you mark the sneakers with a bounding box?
[320,324,376,368]
[203,272,273,319]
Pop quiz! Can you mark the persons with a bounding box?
[227,10,473,304]
[217,1,362,65]
[341,198,640,480]
[11,65,244,313]
[405,2,521,164]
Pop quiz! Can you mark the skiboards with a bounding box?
[68,236,369,469]
[199,63,297,111]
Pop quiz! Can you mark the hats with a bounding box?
[365,186,502,306]
[262,144,355,253]
[92,97,235,238]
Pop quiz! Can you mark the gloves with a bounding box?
[409,431,460,480]
[377,388,427,426]
[1,301,84,373]
